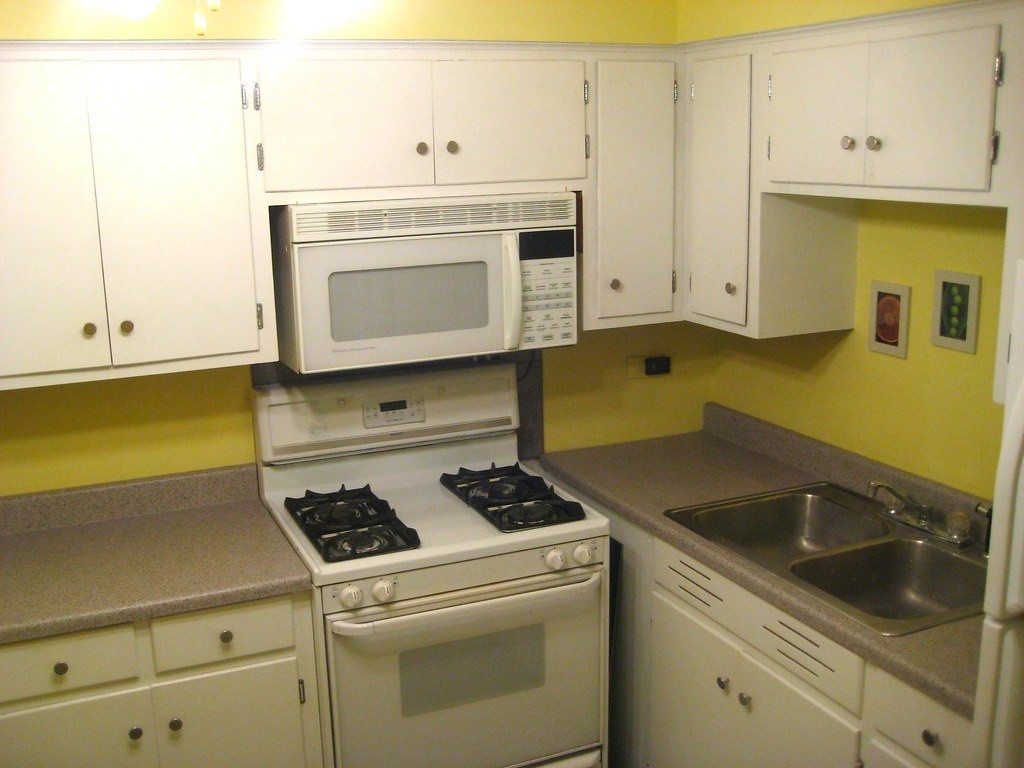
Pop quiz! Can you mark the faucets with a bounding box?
[866,478,937,522]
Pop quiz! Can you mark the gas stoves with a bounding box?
[252,364,612,615]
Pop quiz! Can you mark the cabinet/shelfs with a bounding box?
[583,45,684,336]
[253,44,590,196]
[0,589,327,768]
[680,20,854,340]
[861,660,973,767]
[645,538,867,766]
[609,515,646,767]
[768,2,1023,211]
[1,42,282,395]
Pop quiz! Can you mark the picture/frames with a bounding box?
[867,280,912,360]
[930,266,982,356]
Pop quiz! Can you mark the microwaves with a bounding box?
[276,192,579,374]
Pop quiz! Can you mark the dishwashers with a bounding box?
[315,563,611,768]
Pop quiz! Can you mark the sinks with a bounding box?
[662,479,909,570]
[783,527,998,638]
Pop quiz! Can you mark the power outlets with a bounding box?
[627,356,648,379]
